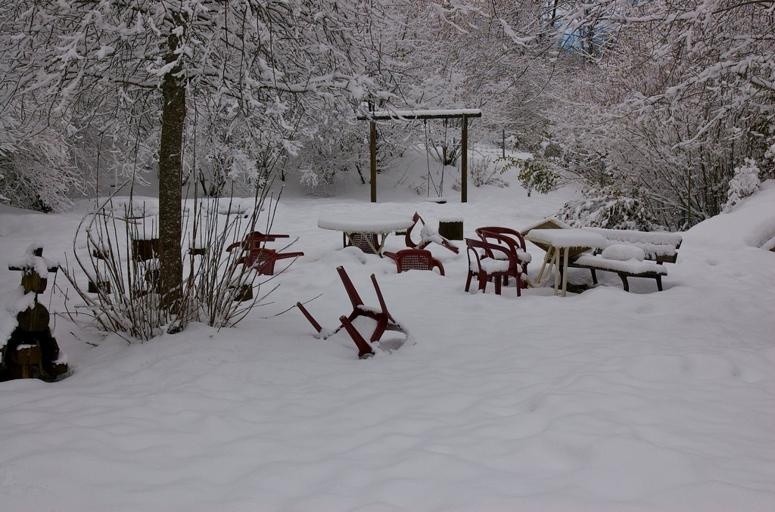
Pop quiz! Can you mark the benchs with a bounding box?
[546,228,684,294]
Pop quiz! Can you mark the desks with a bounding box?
[528,237,593,298]
[315,212,414,259]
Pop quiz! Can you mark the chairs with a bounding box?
[226,229,304,277]
[381,249,446,278]
[295,263,417,359]
[464,225,530,298]
[394,212,461,256]
